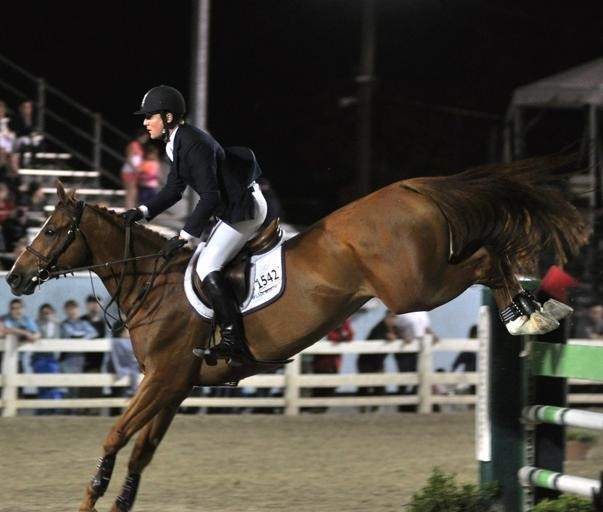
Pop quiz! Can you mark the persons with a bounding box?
[211,317,354,415]
[121,84,267,367]
[357,308,440,415]
[0,98,164,251]
[434,324,477,414]
[0,295,140,416]
[570,297,602,408]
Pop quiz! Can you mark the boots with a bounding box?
[192,271,250,360]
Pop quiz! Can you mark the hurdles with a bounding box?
[476,280,603,512]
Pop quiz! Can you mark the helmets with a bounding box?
[131,84,187,118]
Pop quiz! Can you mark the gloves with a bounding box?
[160,236,188,259]
[119,207,143,223]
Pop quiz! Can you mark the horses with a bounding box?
[5,139,597,512]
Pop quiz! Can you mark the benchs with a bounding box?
[2,147,126,263]
